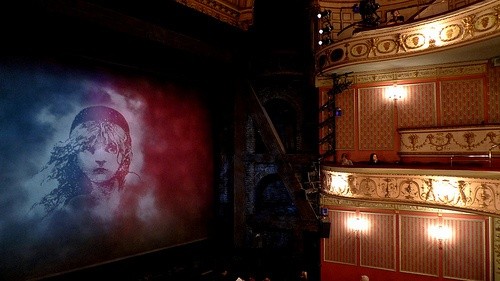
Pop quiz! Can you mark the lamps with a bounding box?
[318,38,334,45]
[320,99,334,113]
[319,150,338,161]
[316,10,332,18]
[319,116,334,129]
[320,133,334,145]
[319,26,333,35]
